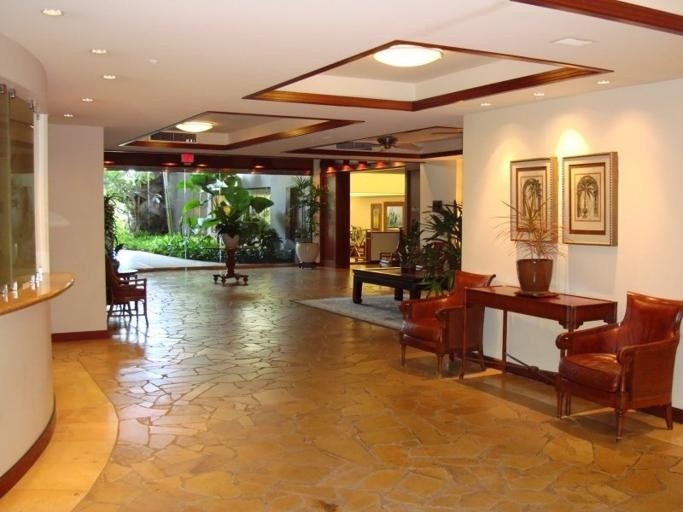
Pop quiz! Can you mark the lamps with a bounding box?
[176,122,213,133]
[181,154,194,164]
[374,45,445,67]
[326,160,407,174]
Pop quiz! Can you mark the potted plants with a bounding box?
[295,175,329,263]
[488,179,571,298]
[397,221,425,274]
[176,171,275,250]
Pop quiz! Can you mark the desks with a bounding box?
[352,267,462,304]
[459,284,618,415]
[117,270,138,317]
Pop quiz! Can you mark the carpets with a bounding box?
[289,291,427,330]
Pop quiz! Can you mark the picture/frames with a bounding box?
[510,157,558,244]
[370,203,382,231]
[561,151,618,247]
[383,201,404,229]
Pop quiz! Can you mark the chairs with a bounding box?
[399,271,497,376]
[106,254,149,328]
[556,291,683,439]
